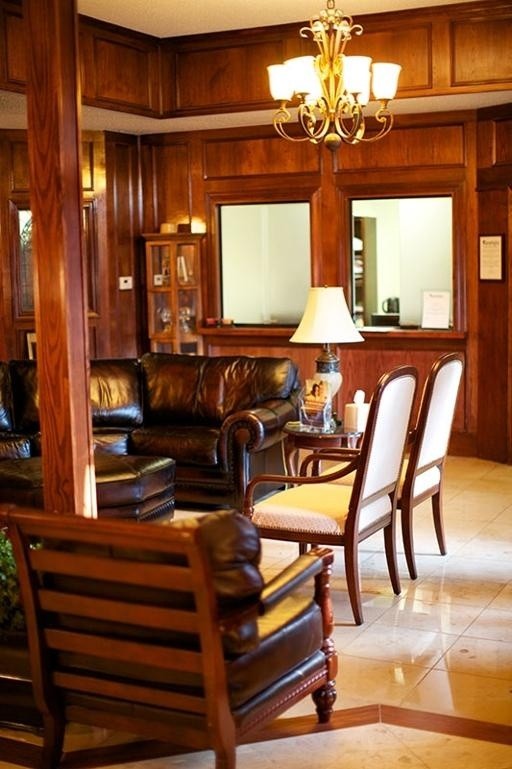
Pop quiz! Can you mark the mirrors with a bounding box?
[9,196,99,325]
[205,186,320,337]
[336,180,467,339]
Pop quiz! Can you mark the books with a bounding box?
[181,255,191,283]
[176,258,181,280]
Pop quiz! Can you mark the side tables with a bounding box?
[283,426,363,488]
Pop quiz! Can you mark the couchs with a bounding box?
[0,356,304,521]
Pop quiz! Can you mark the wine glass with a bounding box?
[178,304,192,334]
[158,305,173,333]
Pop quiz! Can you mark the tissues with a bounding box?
[343,389,370,433]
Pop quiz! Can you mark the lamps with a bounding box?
[289,285,365,427]
[267,0,402,151]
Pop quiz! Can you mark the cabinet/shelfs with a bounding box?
[350,215,377,325]
[143,232,212,356]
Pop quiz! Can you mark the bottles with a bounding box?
[176,256,188,282]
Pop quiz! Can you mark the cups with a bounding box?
[382,297,400,314]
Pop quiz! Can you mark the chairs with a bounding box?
[312,351,465,580]
[0,503,338,769]
[245,365,418,626]
